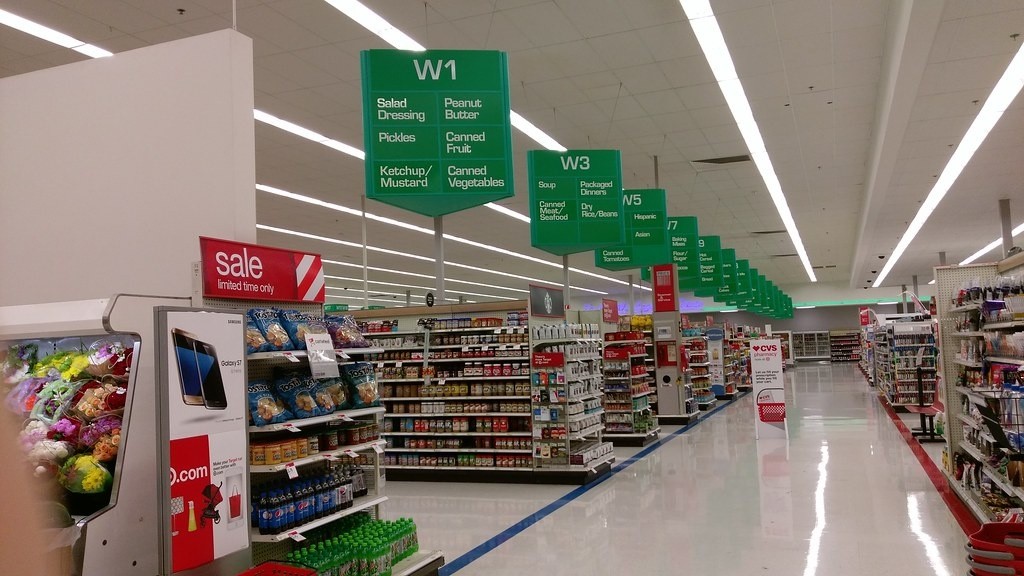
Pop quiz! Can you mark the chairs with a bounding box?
[905,376,946,444]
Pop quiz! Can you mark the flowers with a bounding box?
[4,338,136,515]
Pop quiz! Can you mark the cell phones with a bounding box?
[172,328,205,406]
[193,341,228,410]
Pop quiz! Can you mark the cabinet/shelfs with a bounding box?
[192,249,1024,576]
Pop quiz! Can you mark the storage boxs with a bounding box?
[605,444,608,454]
[603,442,613,452]
[589,441,599,446]
[575,453,588,465]
[599,446,606,455]
[634,423,640,433]
[640,423,648,434]
[569,451,580,464]
[585,449,599,459]
[649,419,655,430]
[583,450,593,461]
[592,447,603,457]
[654,417,658,428]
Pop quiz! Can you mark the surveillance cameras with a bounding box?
[393,297,396,298]
[344,288,347,290]
[878,256,885,259]
[871,271,876,273]
[867,280,871,282]
[864,287,867,289]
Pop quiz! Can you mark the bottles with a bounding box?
[249,462,367,535]
[285,511,419,576]
